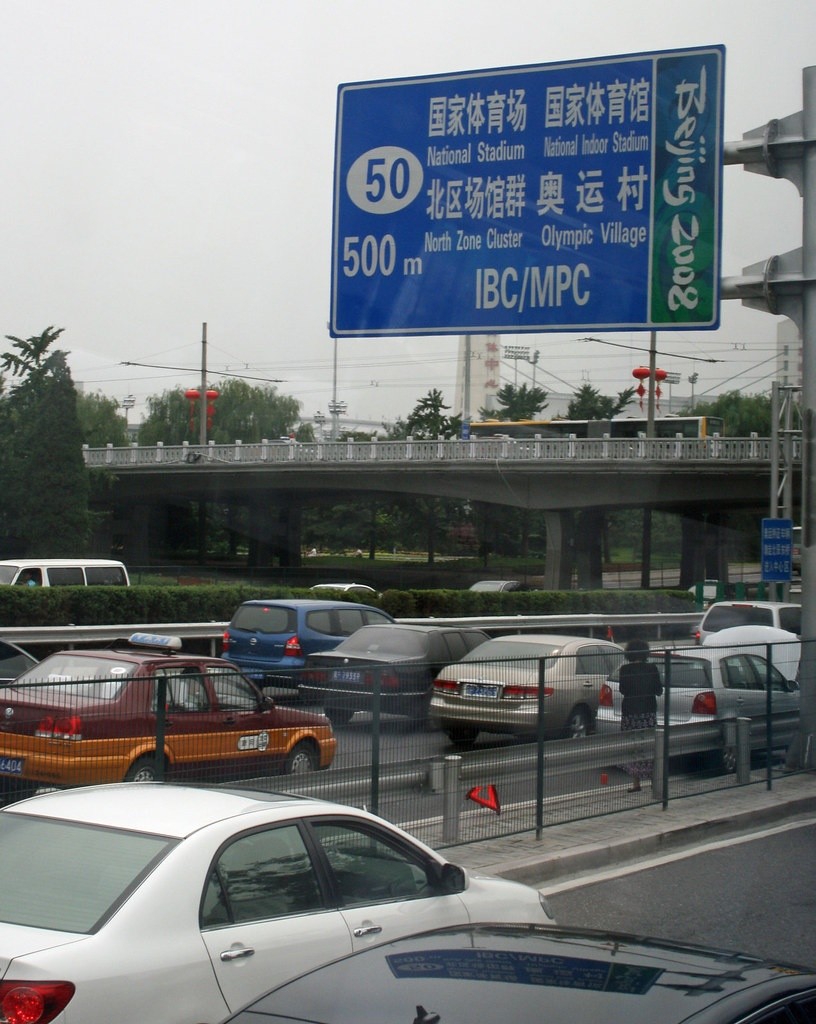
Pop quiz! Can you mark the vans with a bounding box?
[220,599,398,693]
[696,601,802,645]
[0,559,131,587]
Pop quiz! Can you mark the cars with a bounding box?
[297,624,492,724]
[469,581,538,592]
[687,580,718,599]
[218,921,815,1024]
[0,632,337,786]
[428,634,625,747]
[0,781,557,1023]
[309,583,383,596]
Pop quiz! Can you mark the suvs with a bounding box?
[595,645,799,734]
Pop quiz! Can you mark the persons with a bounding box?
[308,546,316,557]
[355,545,362,559]
[180,667,201,705]
[22,571,36,586]
[618,640,663,793]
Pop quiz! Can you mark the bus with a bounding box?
[470,414,724,438]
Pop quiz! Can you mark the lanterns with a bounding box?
[205,390,219,400]
[655,369,667,381]
[632,368,650,380]
[185,389,200,399]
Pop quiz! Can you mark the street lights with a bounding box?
[689,376,697,410]
[122,394,135,438]
[662,372,681,413]
[329,399,347,438]
[314,410,325,438]
[505,346,529,391]
